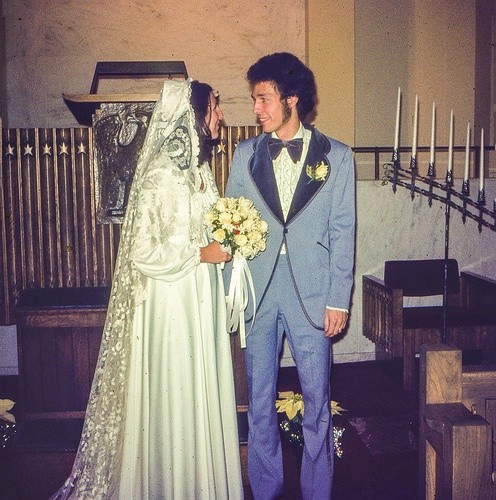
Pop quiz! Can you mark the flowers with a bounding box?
[274,390,349,423]
[0,399,17,425]
[204,195,269,261]
[304,160,328,182]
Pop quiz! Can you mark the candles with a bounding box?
[480,127,485,191]
[412,93,419,158]
[464,120,471,181]
[430,101,435,165]
[448,108,454,172]
[394,86,401,150]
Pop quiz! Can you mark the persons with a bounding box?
[221,51,355,500]
[49,77,244,500]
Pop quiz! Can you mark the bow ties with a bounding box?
[266,138,304,164]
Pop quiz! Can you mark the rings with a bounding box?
[339,328,342,333]
